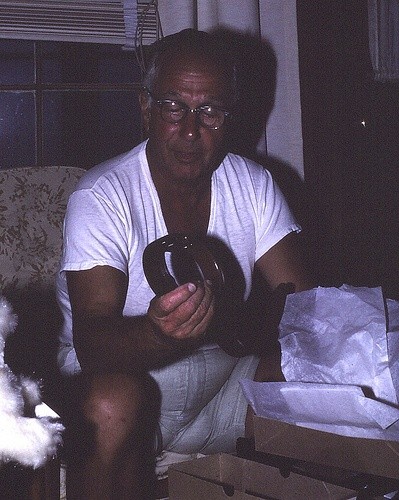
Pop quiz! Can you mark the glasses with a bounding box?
[142,85,238,131]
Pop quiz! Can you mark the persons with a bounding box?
[57,28,320,498]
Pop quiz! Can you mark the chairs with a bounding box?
[0,168,123,500]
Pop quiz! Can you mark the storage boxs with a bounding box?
[252,414,399,480]
[167,453,361,500]
